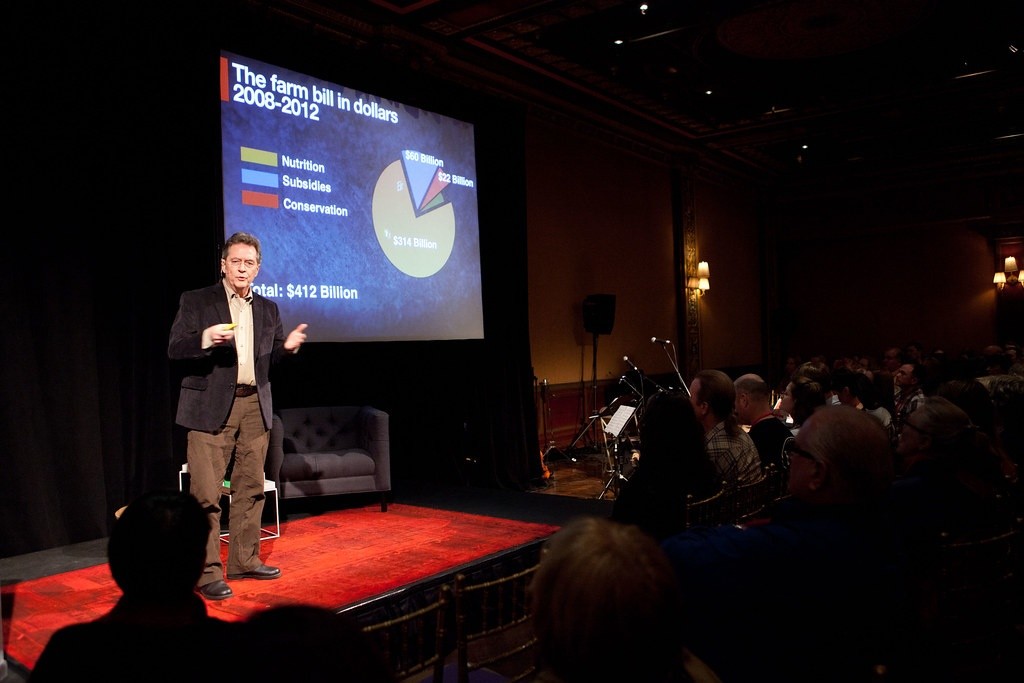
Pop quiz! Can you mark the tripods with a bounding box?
[541,334,630,502]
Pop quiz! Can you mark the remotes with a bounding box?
[224,323,238,331]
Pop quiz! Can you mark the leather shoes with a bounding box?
[226,563,282,580]
[200,580,233,600]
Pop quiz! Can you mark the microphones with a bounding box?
[651,337,672,343]
[623,356,639,372]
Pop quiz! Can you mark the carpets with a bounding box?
[0,502,562,671]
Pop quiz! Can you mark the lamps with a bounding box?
[698,261,710,296]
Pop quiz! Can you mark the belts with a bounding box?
[234,387,257,397]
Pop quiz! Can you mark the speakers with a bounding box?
[583,293,617,336]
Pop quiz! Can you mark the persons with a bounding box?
[521,336,1023,683]
[26,486,400,683]
[166,232,307,599]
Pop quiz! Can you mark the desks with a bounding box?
[178,461,280,543]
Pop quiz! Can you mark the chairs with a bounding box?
[351,549,551,683]
[901,517,1024,683]
[891,398,907,455]
[685,448,791,527]
[262,404,391,522]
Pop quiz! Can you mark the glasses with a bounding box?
[783,437,817,463]
[901,413,930,434]
